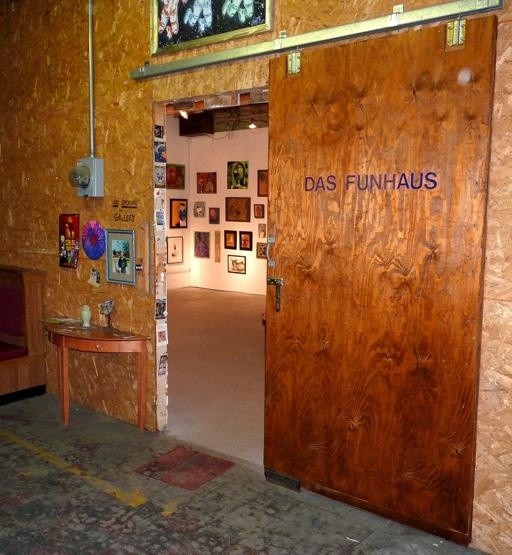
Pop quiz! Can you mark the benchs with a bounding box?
[0,264,48,395]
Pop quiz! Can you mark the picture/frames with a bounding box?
[228,255,246,274]
[166,237,183,264]
[257,170,268,197]
[105,229,136,286]
[150,0,273,57]
[254,204,264,218]
[224,230,237,249]
[256,242,267,258]
[239,231,252,250]
[170,198,187,228]
[225,197,251,222]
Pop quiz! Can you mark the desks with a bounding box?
[45,322,151,432]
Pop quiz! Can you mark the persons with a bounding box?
[117,249,128,273]
